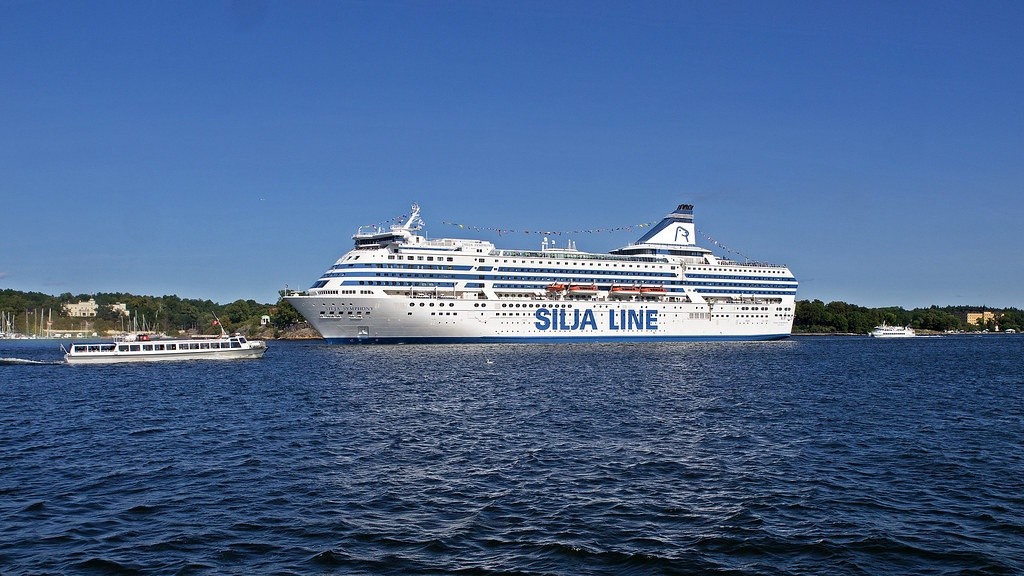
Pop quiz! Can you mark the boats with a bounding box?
[610,285,641,298]
[54,334,62,339]
[544,284,565,291]
[569,286,596,296]
[62,312,269,362]
[867,321,915,338]
[64,333,72,339]
[76,332,84,339]
[640,288,665,298]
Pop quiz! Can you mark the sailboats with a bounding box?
[0,307,55,340]
[113,310,192,340]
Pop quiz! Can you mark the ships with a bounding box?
[278,200,800,346]
